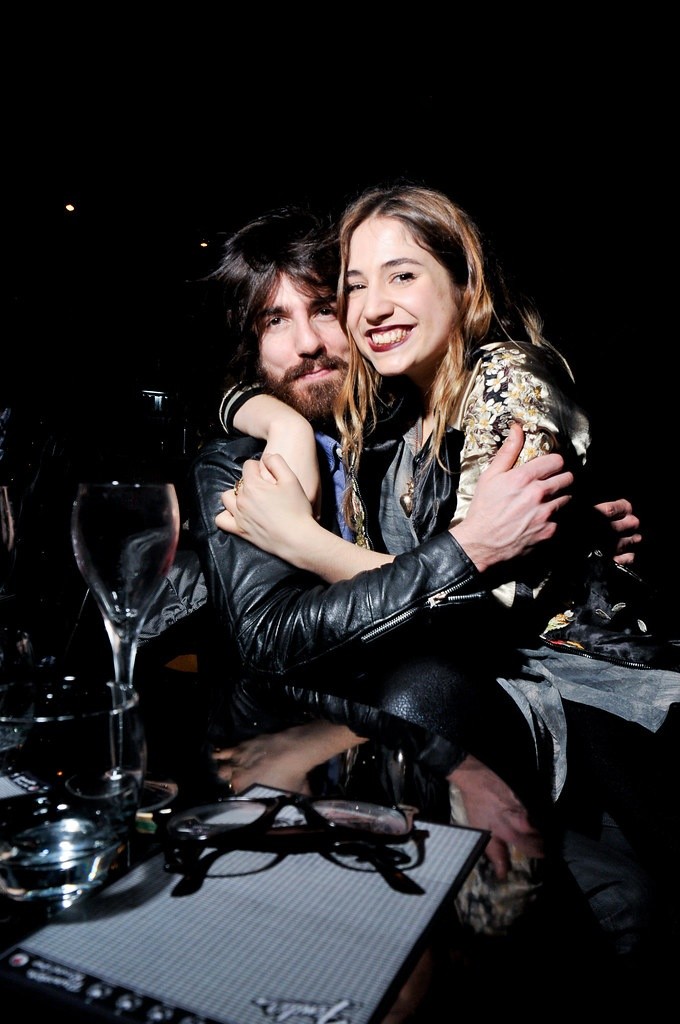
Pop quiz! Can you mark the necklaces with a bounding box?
[400,420,419,518]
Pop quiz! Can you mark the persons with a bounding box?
[204,679,550,1024]
[182,180,680,953]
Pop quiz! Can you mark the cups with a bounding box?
[1,675,139,902]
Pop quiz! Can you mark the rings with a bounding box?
[234,479,243,495]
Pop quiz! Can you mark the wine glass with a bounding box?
[69,478,182,813]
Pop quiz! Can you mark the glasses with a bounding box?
[163,795,428,898]
